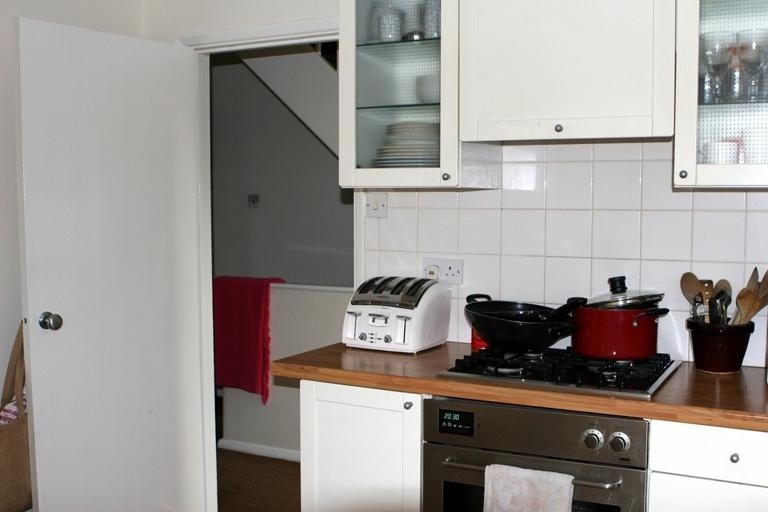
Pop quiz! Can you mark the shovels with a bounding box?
[727,266,768,326]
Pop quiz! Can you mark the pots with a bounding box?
[572,275,668,361]
[464,292,575,358]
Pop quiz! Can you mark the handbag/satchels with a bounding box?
[0,317,34,512]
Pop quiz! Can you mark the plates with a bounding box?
[370,122,438,168]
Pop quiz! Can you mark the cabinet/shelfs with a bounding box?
[299,380,423,512]
[675,0,768,192]
[647,418,768,512]
[340,0,504,189]
[459,0,675,142]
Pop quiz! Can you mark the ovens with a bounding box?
[421,444,647,512]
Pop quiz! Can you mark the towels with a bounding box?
[213,276,286,403]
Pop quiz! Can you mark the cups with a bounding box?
[366,2,439,42]
[687,317,755,378]
[698,128,766,164]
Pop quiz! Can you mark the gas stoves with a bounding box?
[422,344,683,469]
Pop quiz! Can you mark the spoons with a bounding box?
[679,273,767,324]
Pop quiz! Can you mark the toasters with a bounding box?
[341,276,453,355]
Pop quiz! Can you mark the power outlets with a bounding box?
[423,257,463,286]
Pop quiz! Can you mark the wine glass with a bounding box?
[700,30,768,105]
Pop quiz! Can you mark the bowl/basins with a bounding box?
[414,73,440,103]
